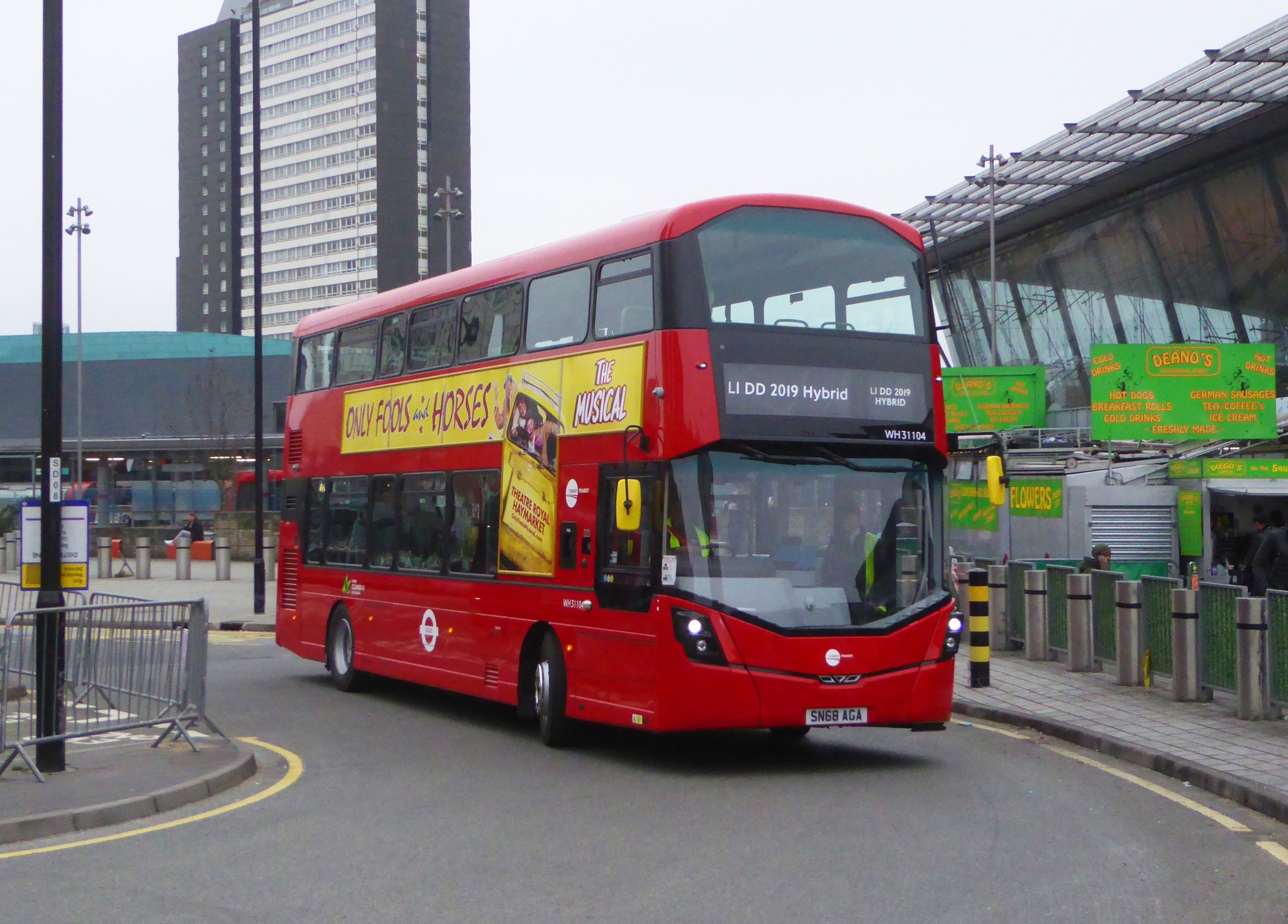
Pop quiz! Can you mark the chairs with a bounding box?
[619,303,652,335]
[336,355,453,384]
[773,319,855,332]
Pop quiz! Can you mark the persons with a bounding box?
[166,512,203,546]
[822,502,894,625]
[647,500,709,586]
[1079,543,1111,574]
[1239,510,1288,595]
[493,375,559,467]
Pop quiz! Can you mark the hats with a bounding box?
[1092,544,1111,557]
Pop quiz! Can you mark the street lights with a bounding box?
[64,197,93,500]
[433,176,464,273]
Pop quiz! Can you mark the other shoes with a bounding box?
[164,540,174,546]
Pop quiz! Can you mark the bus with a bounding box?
[277,196,1012,749]
[64,479,234,526]
[232,470,282,528]
